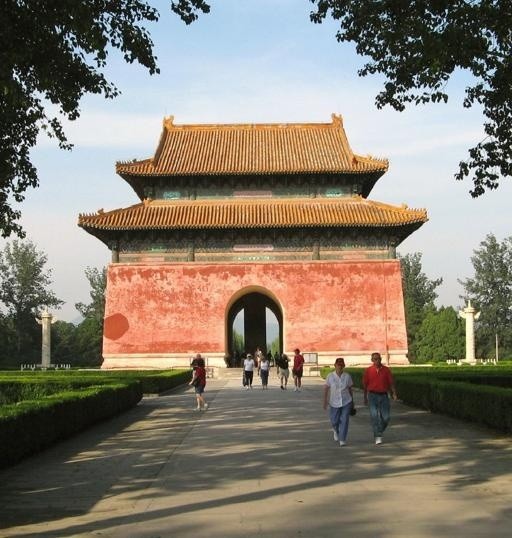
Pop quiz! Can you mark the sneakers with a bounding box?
[192,403,209,412]
[340,441,346,446]
[376,437,382,444]
[333,430,340,440]
[280,386,303,392]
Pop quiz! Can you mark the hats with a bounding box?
[334,358,345,368]
[294,349,301,353]
[247,354,252,358]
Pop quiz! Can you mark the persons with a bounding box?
[323,359,354,446]
[362,352,397,445]
[188,354,208,412]
[224,348,304,392]
